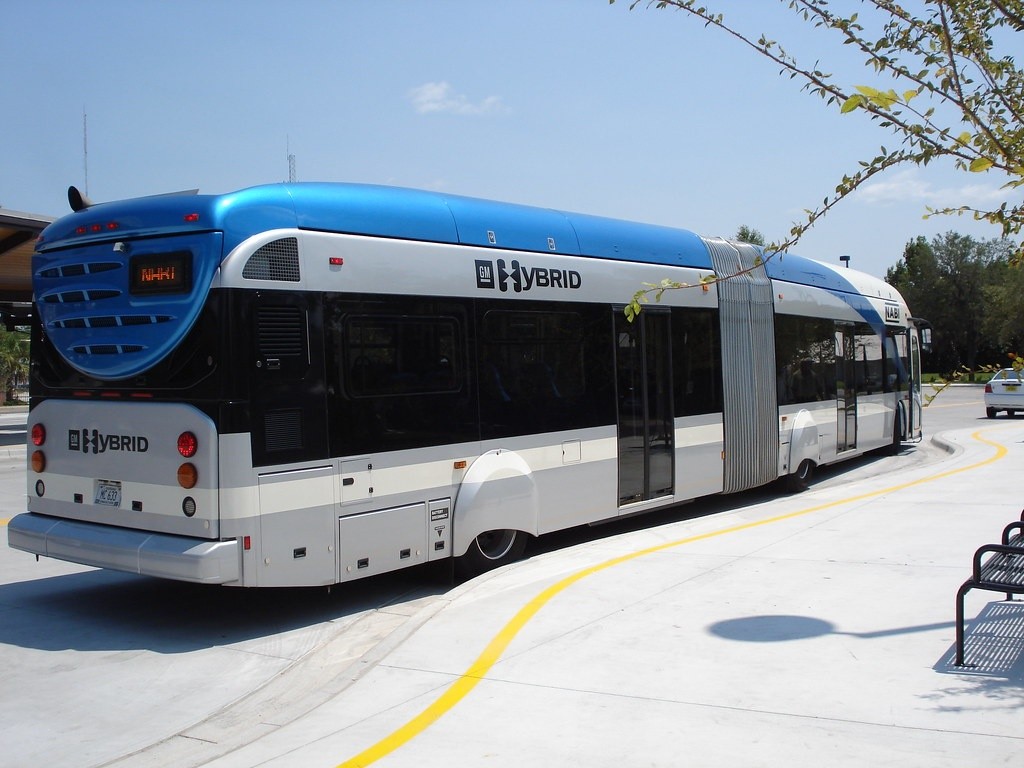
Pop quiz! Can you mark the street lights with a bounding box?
[839,255,852,268]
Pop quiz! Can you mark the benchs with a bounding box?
[954,510,1024,667]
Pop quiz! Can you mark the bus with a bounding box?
[6,181,936,587]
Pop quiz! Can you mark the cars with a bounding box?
[984,367,1024,419]
